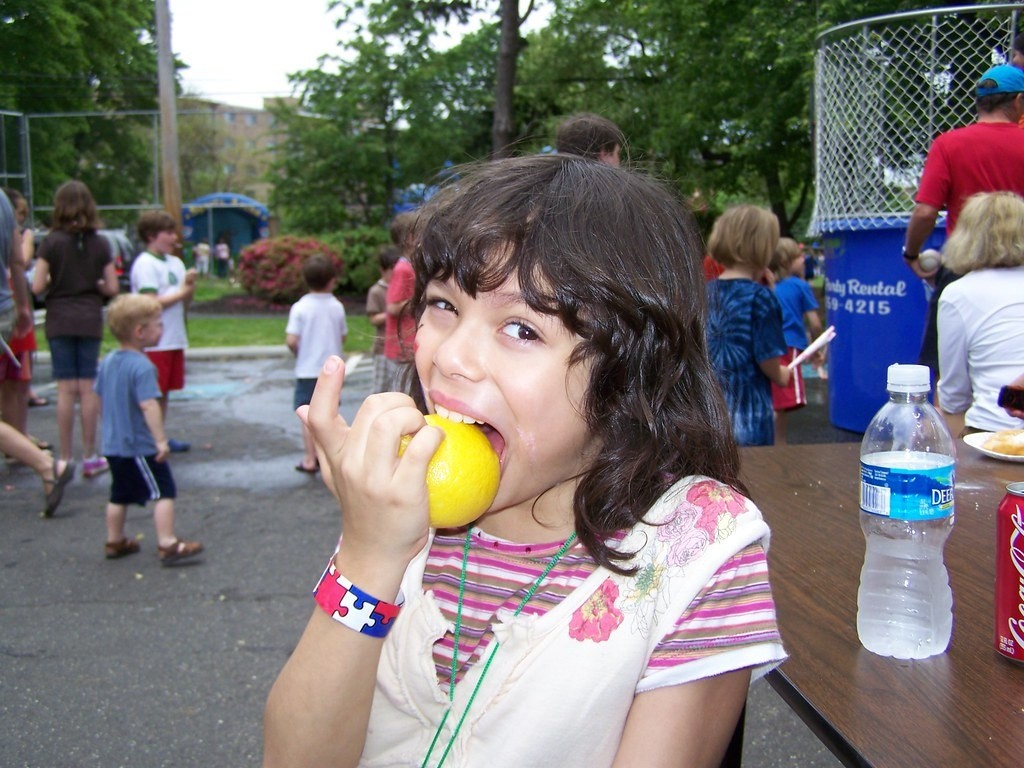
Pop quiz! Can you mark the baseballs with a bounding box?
[920,248,940,272]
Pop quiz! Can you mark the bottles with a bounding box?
[856,363,954,661]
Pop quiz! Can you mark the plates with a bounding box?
[962,431,1023,464]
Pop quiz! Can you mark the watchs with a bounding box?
[903,245,918,260]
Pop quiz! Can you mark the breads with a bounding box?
[983,428,1024,456]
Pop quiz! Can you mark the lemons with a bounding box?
[398,414,502,528]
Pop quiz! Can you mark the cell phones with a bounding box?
[998,386,1024,412]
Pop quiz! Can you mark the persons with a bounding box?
[214,237,230,280]
[1012,31,1024,70]
[937,191,1024,453]
[902,63,1024,441]
[555,110,622,168]
[285,253,348,475]
[129,211,197,452]
[702,202,826,448]
[31,180,120,478]
[192,237,211,280]
[998,373,1024,420]
[246,153,790,768]
[91,292,201,565]
[367,209,424,397]
[0,183,77,519]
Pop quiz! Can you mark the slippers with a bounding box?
[5,450,53,468]
[297,461,318,472]
[28,434,55,451]
[28,394,48,406]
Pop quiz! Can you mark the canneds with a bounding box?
[993,482,1024,666]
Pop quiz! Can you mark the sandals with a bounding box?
[42,456,74,518]
[159,540,203,567]
[107,535,140,558]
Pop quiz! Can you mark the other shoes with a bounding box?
[167,438,191,452]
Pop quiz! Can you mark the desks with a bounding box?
[719,440,1023,768]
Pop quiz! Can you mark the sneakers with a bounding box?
[83,456,110,476]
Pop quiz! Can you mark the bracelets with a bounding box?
[315,553,403,641]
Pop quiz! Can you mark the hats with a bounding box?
[975,64,1024,98]
